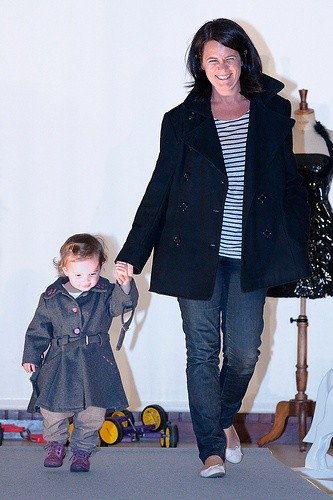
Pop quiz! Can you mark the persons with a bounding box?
[113,18,312,478]
[266,108,333,300]
[23,233,140,474]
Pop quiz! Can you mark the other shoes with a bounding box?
[222,424,243,464]
[200,458,226,478]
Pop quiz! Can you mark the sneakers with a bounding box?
[44,441,67,467]
[68,444,90,473]
